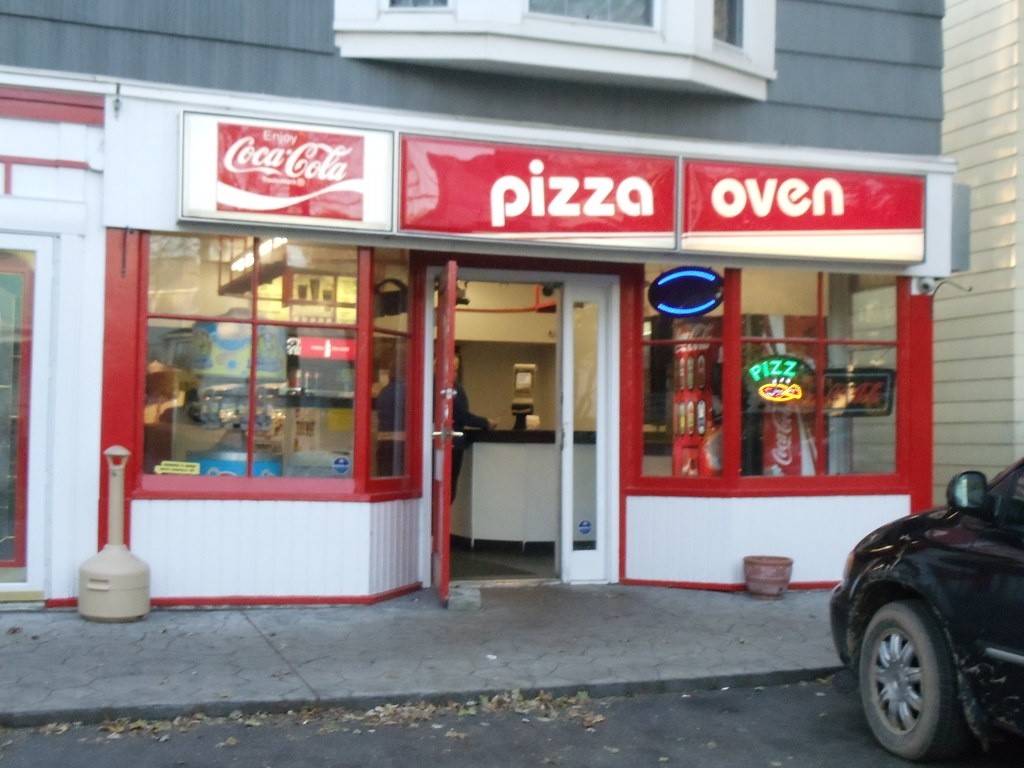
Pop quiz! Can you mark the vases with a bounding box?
[744,555,793,599]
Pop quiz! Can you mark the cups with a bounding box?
[525,415,540,431]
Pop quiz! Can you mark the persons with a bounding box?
[376,358,406,477]
[430,354,499,555]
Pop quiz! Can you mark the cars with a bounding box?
[829,457,1024,768]
